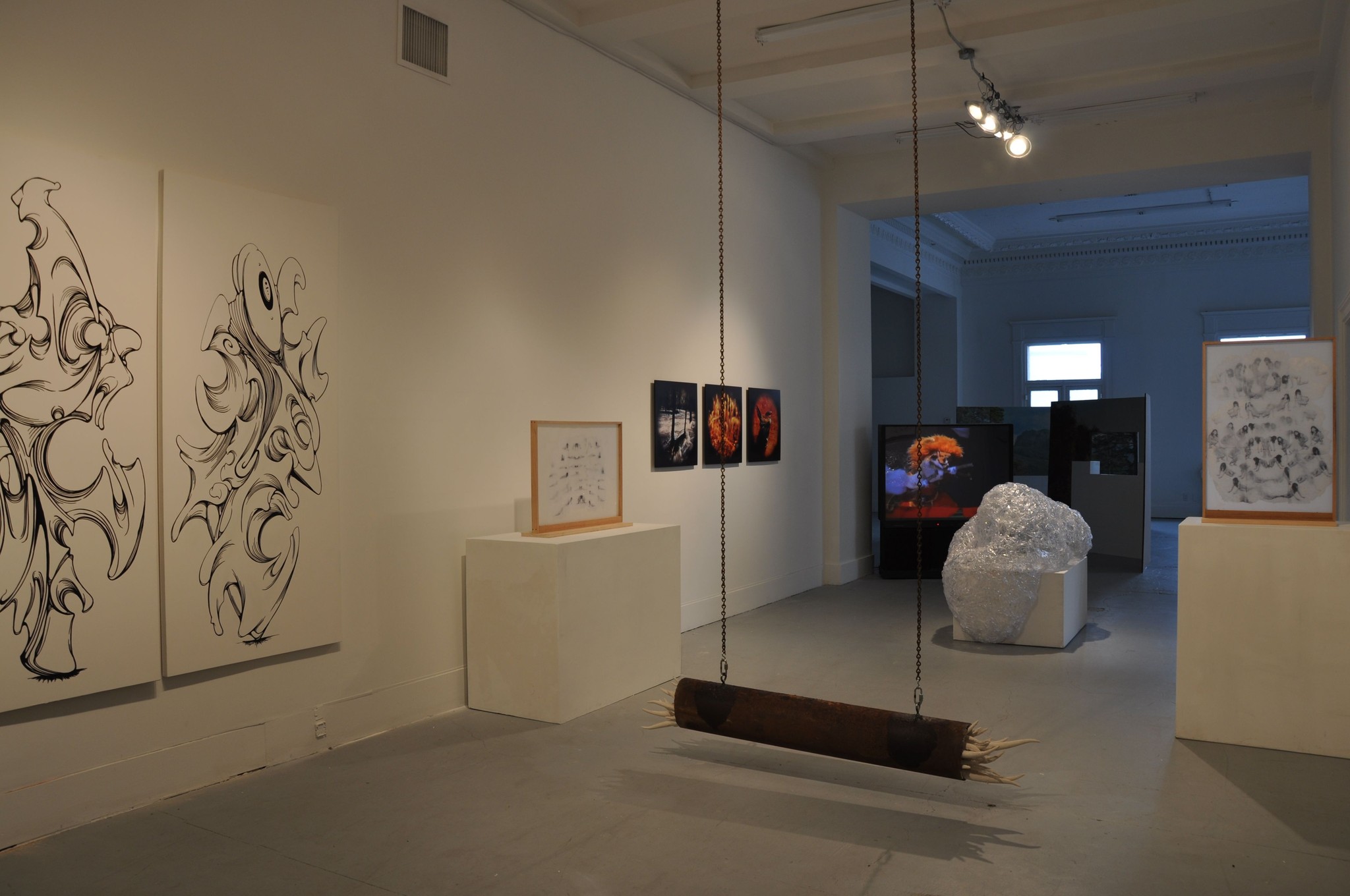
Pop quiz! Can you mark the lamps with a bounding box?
[964,80,1032,158]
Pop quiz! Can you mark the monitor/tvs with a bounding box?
[879,424,1013,524]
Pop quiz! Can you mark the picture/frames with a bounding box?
[1201,337,1335,521]
[530,420,623,534]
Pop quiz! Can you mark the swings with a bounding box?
[641,0,1041,789]
